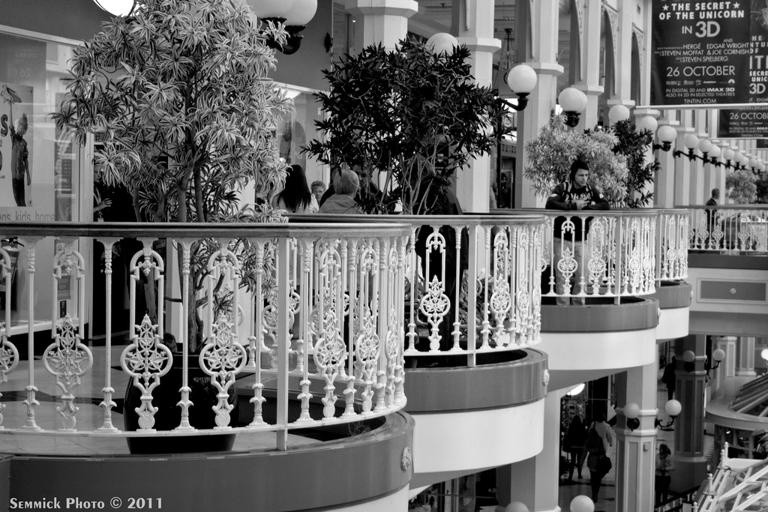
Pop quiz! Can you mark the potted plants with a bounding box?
[719,171,768,248]
[51,0,513,453]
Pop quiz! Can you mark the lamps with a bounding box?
[90,0,768,177]
[505,349,768,512]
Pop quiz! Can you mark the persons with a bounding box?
[583,426,607,504]
[704,187,721,232]
[544,159,612,307]
[318,169,368,215]
[396,166,470,350]
[661,355,677,402]
[309,180,327,202]
[495,173,510,206]
[269,164,320,215]
[587,409,614,454]
[91,164,114,223]
[319,160,396,213]
[654,443,676,507]
[7,114,32,207]
[566,414,589,480]
